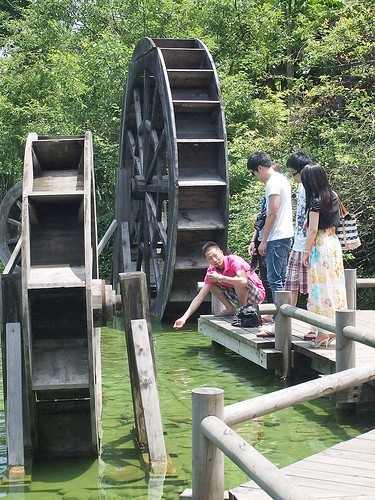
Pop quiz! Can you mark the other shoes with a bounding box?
[257,329,275,338]
[304,332,316,340]
[214,309,237,318]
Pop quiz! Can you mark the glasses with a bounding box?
[251,164,262,176]
[291,172,298,177]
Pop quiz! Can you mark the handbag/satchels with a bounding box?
[232,304,262,327]
[332,190,361,250]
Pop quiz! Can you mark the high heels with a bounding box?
[320,336,336,346]
[310,337,330,348]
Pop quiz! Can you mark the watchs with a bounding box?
[216,275,223,283]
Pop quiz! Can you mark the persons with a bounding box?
[247,151,348,349]
[173,241,266,330]
